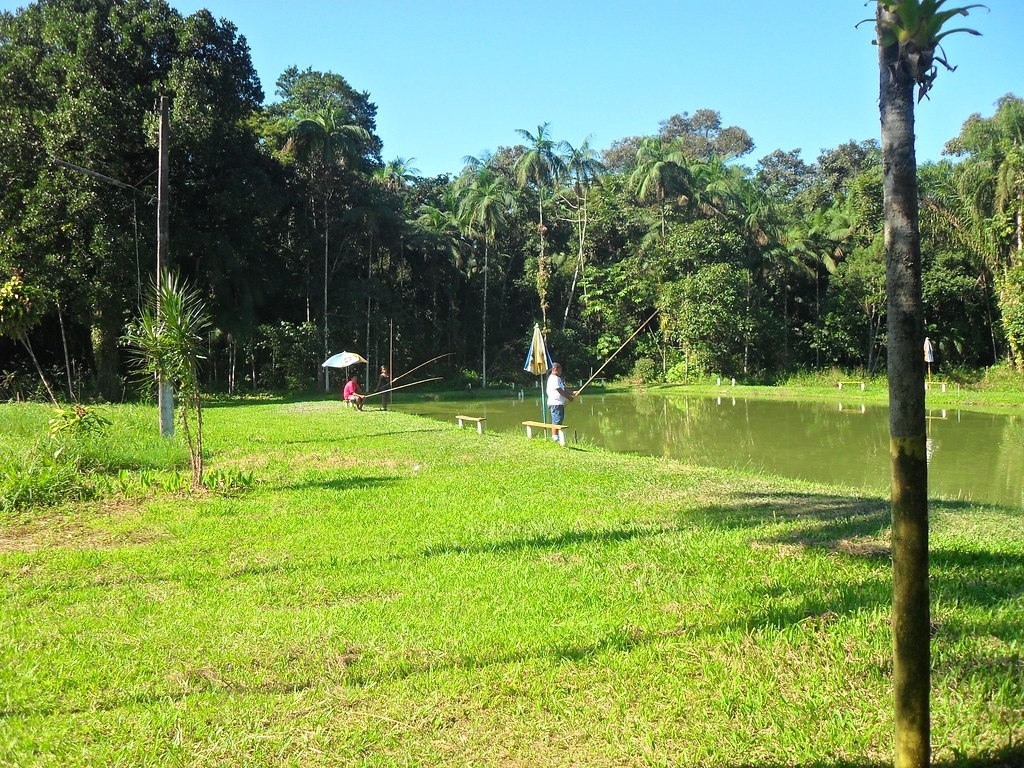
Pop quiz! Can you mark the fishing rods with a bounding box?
[365,377,444,399]
[379,352,456,390]
[564,307,661,408]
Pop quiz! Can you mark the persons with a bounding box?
[547,363,578,441]
[376,365,390,411]
[344,375,365,411]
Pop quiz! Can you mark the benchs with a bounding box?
[925,410,948,419]
[925,382,947,392]
[838,382,865,391]
[455,415,486,435]
[578,377,607,387]
[344,399,355,408]
[838,402,865,414]
[522,420,569,446]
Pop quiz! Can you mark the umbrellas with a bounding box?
[524,323,553,424]
[322,351,369,379]
[924,337,934,382]
